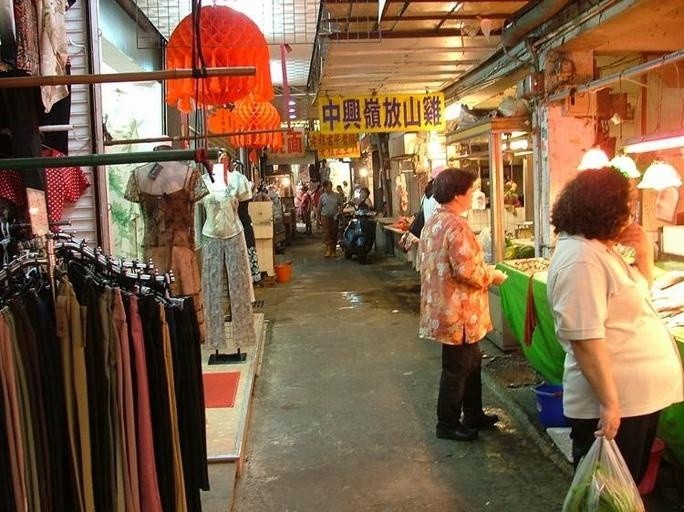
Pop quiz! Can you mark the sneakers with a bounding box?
[303,230,311,234]
[462,411,498,427]
[435,423,478,440]
[324,250,336,257]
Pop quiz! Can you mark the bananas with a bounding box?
[567,462,635,512]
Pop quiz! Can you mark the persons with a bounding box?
[124,142,211,346]
[543,161,684,492]
[409,177,446,223]
[290,180,373,259]
[193,161,260,359]
[229,153,263,285]
[414,165,510,442]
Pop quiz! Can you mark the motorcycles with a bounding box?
[336,199,389,265]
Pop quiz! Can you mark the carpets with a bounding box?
[202,373,241,408]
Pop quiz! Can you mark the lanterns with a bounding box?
[161,5,277,114]
[224,100,287,151]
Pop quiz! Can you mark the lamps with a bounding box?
[578,55,684,191]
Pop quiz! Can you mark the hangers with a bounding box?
[1,230,174,307]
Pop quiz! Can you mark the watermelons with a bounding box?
[504,243,534,260]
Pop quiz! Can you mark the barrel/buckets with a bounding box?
[531,383,567,428]
